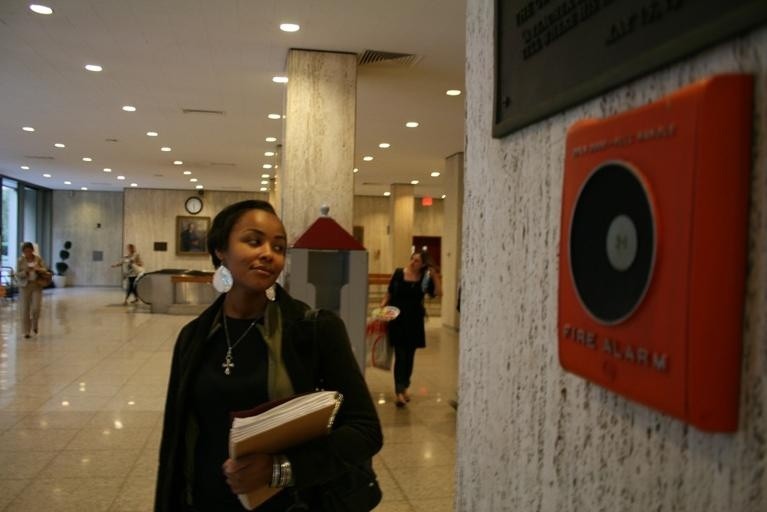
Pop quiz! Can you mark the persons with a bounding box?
[378,249,441,408]
[12,240,47,339]
[179,222,200,253]
[109,243,144,306]
[152,200,386,512]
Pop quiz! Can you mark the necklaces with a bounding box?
[221,293,261,376]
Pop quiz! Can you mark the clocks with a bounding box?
[185,197,203,215]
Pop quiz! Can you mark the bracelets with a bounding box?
[266,453,293,488]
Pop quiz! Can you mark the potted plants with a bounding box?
[52,241,73,288]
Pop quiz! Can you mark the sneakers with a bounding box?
[396,399,410,407]
[25,329,37,338]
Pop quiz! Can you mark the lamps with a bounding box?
[421,198,433,206]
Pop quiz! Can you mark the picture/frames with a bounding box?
[490,0,767,139]
[176,215,211,256]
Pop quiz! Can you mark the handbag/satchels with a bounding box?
[366,317,393,371]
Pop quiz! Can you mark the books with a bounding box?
[226,388,343,510]
